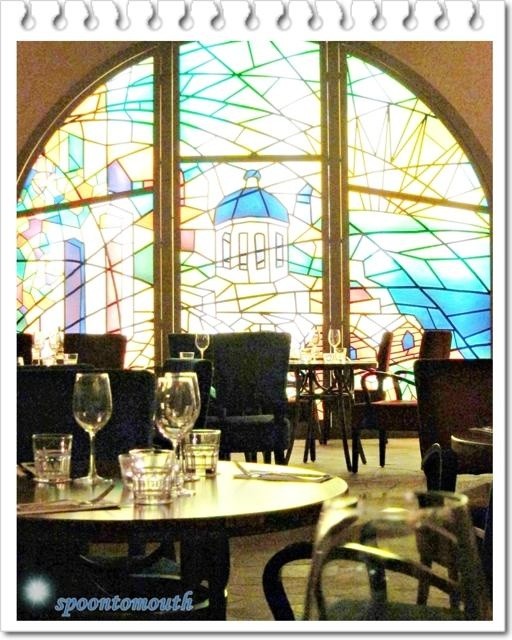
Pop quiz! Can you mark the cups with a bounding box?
[74,374,111,485]
[157,378,194,495]
[329,329,339,353]
[181,430,217,478]
[130,446,175,505]
[119,454,133,488]
[169,371,196,482]
[31,434,70,481]
[196,332,210,358]
[334,348,346,361]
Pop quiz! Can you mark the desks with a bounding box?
[289,358,378,464]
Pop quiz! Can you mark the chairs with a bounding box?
[262,492,493,621]
[16,331,35,367]
[18,366,157,464]
[162,358,213,450]
[414,358,493,492]
[168,333,291,463]
[349,328,452,474]
[303,332,393,464]
[63,332,125,366]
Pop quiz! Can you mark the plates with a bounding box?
[22,462,71,483]
